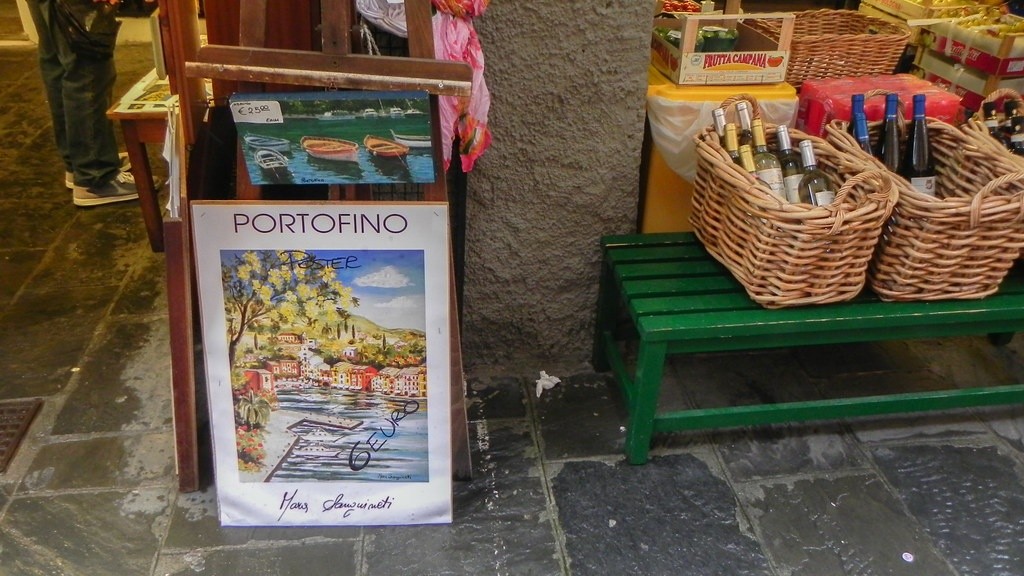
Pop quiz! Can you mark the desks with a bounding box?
[105,33,214,252]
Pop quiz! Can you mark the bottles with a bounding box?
[798,140,837,208]
[854,112,874,156]
[724,123,739,165]
[712,108,726,147]
[751,118,787,200]
[736,102,754,155]
[983,100,1024,157]
[878,94,905,176]
[905,94,935,197]
[847,94,864,137]
[775,125,805,204]
[738,145,772,190]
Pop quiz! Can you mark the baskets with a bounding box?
[825,117,1024,302]
[961,88,1024,168]
[688,115,897,308]
[746,8,913,88]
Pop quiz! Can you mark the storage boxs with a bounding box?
[858,0,996,64]
[910,12,1024,121]
[652,12,796,87]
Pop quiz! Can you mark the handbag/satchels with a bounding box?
[54,0,119,60]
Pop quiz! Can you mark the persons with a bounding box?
[27,0,161,206]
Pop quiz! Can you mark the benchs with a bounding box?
[590,234,1024,465]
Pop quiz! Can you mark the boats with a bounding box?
[241,132,292,153]
[363,134,409,158]
[253,146,290,178]
[300,135,362,164]
[390,128,433,151]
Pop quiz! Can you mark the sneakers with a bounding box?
[72,173,163,207]
[65,152,132,189]
